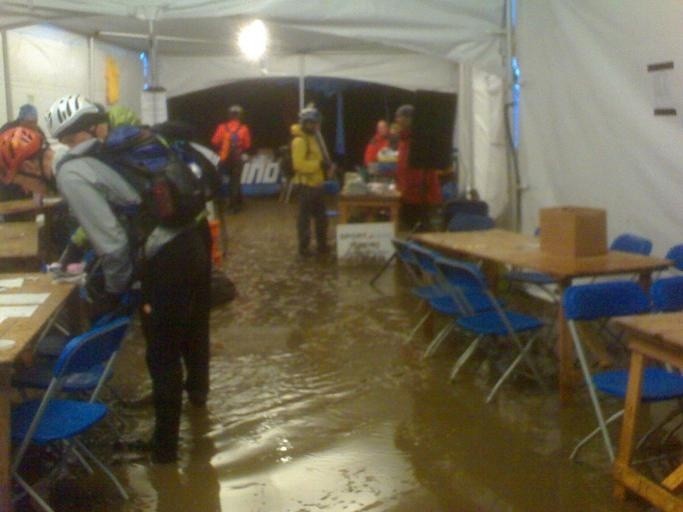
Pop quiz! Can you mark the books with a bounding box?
[538,207,608,258]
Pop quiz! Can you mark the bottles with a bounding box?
[230,105,243,114]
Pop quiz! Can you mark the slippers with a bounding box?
[277,136,294,174]
[226,134,242,168]
[97,122,204,225]
[169,137,220,199]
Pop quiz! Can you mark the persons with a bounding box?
[0,123,195,463]
[290,106,336,255]
[392,104,442,238]
[5,104,46,137]
[364,117,391,164]
[46,95,212,409]
[211,105,250,212]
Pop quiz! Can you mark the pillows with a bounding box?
[0,119,44,186]
[46,93,102,139]
[299,109,321,121]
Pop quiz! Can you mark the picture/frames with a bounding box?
[415,228,666,385]
[337,183,403,233]
[1,268,82,507]
[0,218,45,268]
[606,310,683,511]
[1,195,66,219]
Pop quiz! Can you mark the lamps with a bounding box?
[437,254,551,404]
[12,301,129,474]
[662,245,683,274]
[650,274,683,464]
[447,211,495,279]
[9,316,132,512]
[411,244,509,369]
[560,276,682,467]
[393,238,469,357]
[562,234,650,359]
[417,201,489,233]
